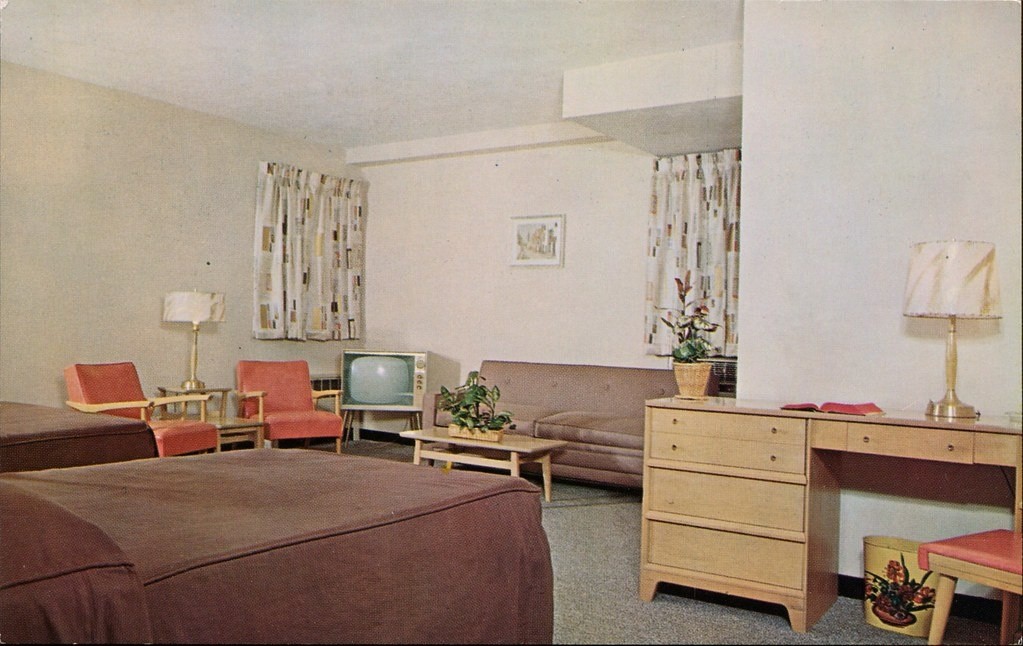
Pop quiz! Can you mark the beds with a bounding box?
[0,399,159,474]
[0,448,554,646]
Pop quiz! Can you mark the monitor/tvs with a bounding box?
[341,349,428,412]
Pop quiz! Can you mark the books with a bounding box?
[779,399,887,415]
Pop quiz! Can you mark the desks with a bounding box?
[639,396,1023,646]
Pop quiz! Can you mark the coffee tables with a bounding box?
[400,427,569,503]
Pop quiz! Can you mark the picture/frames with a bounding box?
[508,213,565,268]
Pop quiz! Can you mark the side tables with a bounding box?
[157,385,269,453]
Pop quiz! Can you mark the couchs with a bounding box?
[64,361,221,458]
[422,359,680,487]
[231,360,343,455]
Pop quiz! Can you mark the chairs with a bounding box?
[917,503,1023,646]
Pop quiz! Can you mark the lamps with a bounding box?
[162,287,226,388]
[903,239,1001,418]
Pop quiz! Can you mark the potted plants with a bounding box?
[438,368,515,442]
[650,267,719,397]
